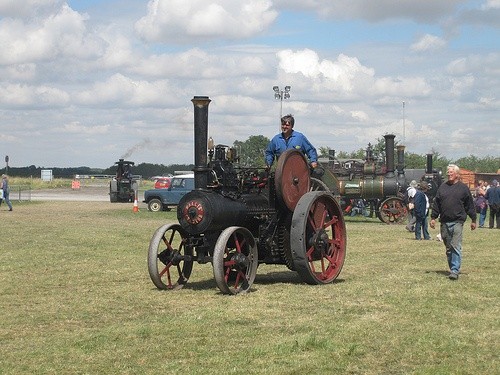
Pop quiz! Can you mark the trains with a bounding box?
[315,133,442,225]
[148,94,347,297]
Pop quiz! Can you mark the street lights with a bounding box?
[272,85,292,118]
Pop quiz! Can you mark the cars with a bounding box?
[154,177,171,190]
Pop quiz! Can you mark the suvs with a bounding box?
[143,173,196,212]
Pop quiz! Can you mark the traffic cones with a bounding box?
[132,198,140,212]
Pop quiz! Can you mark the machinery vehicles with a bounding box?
[108,159,138,203]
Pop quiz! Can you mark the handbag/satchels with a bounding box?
[0,188,4,199]
[409,203,414,209]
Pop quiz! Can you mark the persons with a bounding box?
[0,174,13,212]
[474,179,499,229]
[412,181,431,240]
[264,114,317,170]
[430,164,478,280]
[406,179,417,232]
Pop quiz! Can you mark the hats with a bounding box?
[493,180,497,183]
[410,180,418,187]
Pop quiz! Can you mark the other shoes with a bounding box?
[418,238,423,240]
[449,273,457,279]
[10,208,12,211]
[428,238,433,240]
[406,226,411,231]
